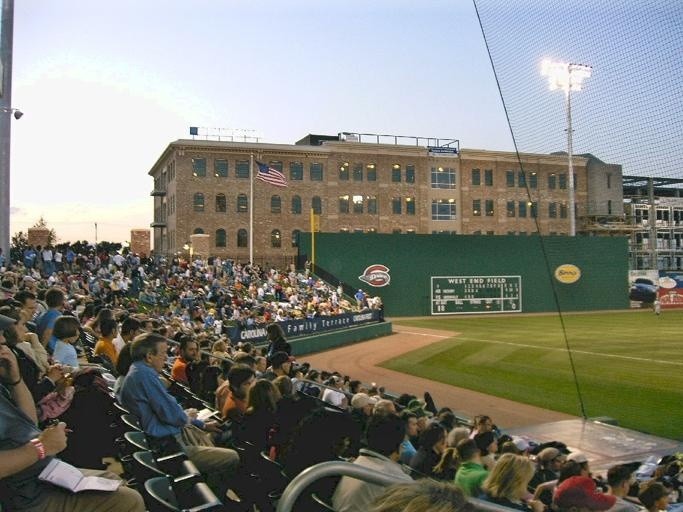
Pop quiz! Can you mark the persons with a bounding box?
[239,379,334,502]
[333,412,682,510]
[0,240,437,411]
[653,298,660,315]
[122,333,239,502]
[0,314,145,512]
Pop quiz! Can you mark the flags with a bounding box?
[255,160,286,189]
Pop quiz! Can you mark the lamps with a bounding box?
[0,106,23,119]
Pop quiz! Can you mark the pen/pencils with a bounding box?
[186,411,200,413]
[44,425,73,433]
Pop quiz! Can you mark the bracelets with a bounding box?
[30,438,44,459]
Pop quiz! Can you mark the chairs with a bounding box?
[0,243,683,512]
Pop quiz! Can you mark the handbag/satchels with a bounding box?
[38,386,75,419]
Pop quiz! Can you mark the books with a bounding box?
[37,457,122,492]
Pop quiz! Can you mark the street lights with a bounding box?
[538,58,595,236]
[182,241,193,278]
[0,107,24,267]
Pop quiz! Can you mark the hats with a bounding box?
[538,447,566,463]
[447,426,472,447]
[564,451,588,465]
[638,481,673,505]
[512,438,530,452]
[23,275,36,283]
[1,314,17,331]
[270,352,296,366]
[397,393,434,418]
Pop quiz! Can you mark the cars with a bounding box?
[629,277,657,303]
